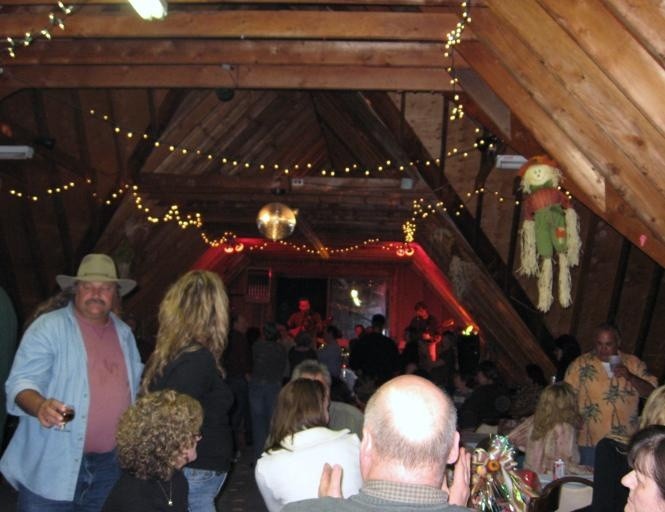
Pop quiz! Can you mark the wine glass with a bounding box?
[54,406,75,432]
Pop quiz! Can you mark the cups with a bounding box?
[608,355,622,372]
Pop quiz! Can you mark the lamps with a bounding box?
[0,145,34,160]
[127,0,168,21]
[496,153,526,169]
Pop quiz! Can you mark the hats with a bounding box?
[55,254,138,298]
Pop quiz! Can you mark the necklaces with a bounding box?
[157,479,173,507]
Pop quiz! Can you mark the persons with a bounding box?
[509,323,665,512]
[254,378,364,512]
[0,284,18,445]
[517,157,583,312]
[279,374,475,511]
[140,269,234,512]
[0,254,145,512]
[102,389,204,512]
[223,299,511,457]
[123,313,152,364]
